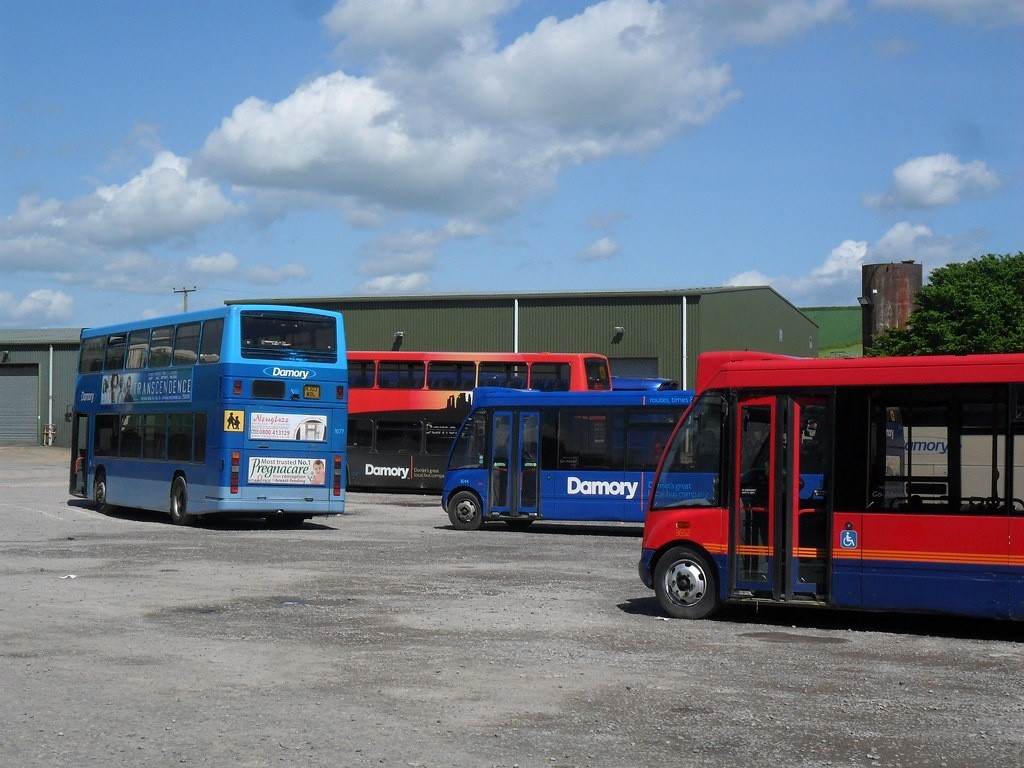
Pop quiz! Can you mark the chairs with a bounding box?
[91,340,291,369]
[899,495,1016,514]
[111,429,204,463]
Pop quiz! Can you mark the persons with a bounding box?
[554,428,584,466]
[311,460,324,484]
[101,375,134,402]
[74,446,87,495]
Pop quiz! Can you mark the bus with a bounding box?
[352,374,680,474]
[637,352,1024,621]
[439,387,910,530]
[345,351,613,492]
[65,304,347,526]
